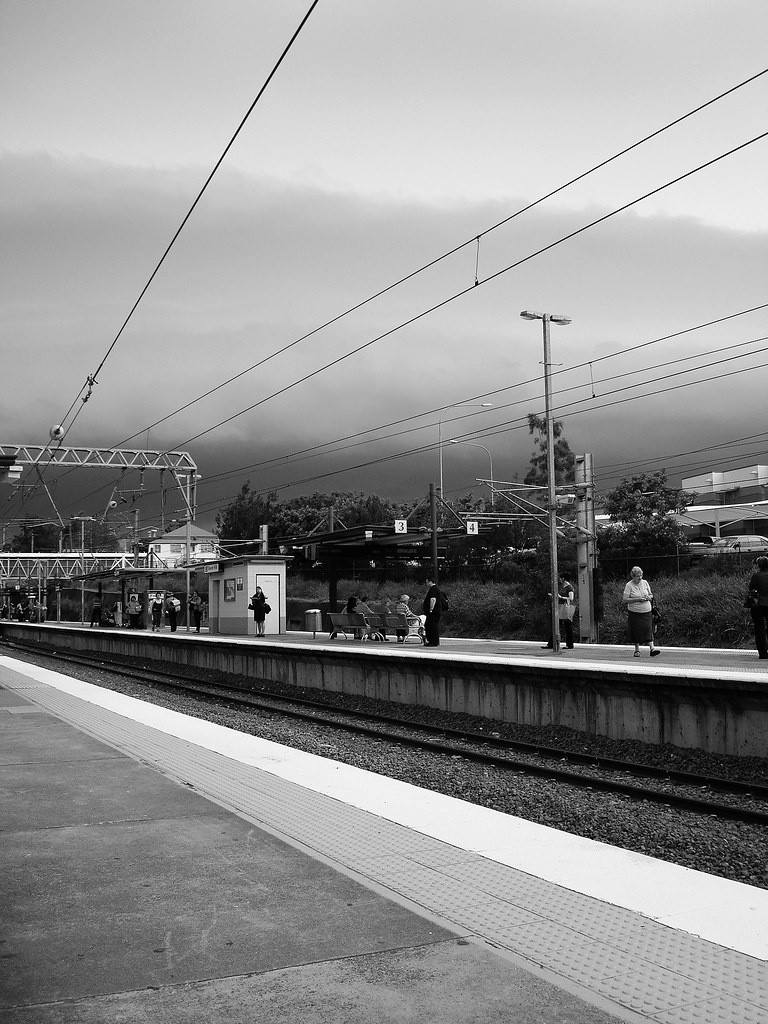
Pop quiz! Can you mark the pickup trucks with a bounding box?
[679,534,723,555]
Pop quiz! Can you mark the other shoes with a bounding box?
[425,642,440,646]
[634,651,640,657]
[563,646,573,649]
[650,648,660,656]
[541,645,552,649]
[193,630,199,633]
[152,626,154,631]
[255,634,266,637]
[375,638,389,641]
[399,639,409,642]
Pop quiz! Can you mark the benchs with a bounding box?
[327,612,385,642]
[360,613,423,645]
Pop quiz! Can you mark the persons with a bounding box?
[251,587,267,637]
[749,555,768,659]
[88,590,202,634]
[540,572,575,649]
[423,576,443,647]
[2,599,49,623]
[622,566,661,657]
[341,593,427,643]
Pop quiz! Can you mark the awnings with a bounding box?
[72,566,195,582]
[277,525,436,565]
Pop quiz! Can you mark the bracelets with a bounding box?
[429,609,433,610]
[639,597,642,602]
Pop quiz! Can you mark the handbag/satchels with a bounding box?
[743,590,759,608]
[135,604,141,611]
[650,595,662,624]
[559,598,575,622]
[265,603,271,614]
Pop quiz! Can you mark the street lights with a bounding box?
[438,403,494,527]
[519,308,574,653]
[448,439,495,512]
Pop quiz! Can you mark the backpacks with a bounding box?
[434,586,449,611]
[171,600,181,613]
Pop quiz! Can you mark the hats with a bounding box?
[166,591,174,597]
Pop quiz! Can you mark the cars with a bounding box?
[703,534,768,556]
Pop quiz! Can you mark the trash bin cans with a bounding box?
[303,609,322,631]
